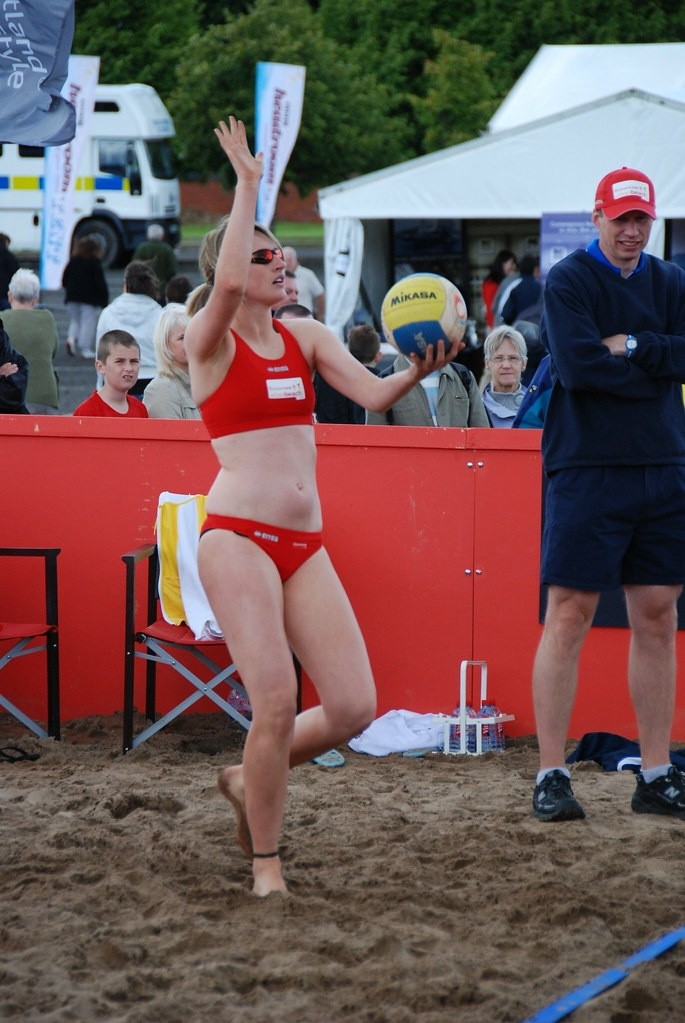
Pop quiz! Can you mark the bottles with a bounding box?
[450,700,505,755]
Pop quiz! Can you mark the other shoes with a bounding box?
[67,339,76,355]
[82,350,95,357]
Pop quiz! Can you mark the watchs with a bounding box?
[625,334,638,358]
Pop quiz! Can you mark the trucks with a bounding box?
[0,83,182,268]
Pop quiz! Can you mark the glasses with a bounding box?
[488,355,523,365]
[249,247,285,265]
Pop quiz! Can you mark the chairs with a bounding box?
[121,547,301,755]
[0,548,64,760]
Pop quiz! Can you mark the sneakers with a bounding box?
[534,766,585,823]
[631,771,685,817]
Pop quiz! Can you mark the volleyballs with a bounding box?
[380,271,468,359]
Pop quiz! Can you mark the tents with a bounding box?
[316,89,684,349]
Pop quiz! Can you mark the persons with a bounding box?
[1,223,558,431]
[178,115,468,896]
[529,168,685,819]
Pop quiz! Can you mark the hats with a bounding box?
[594,166,656,221]
